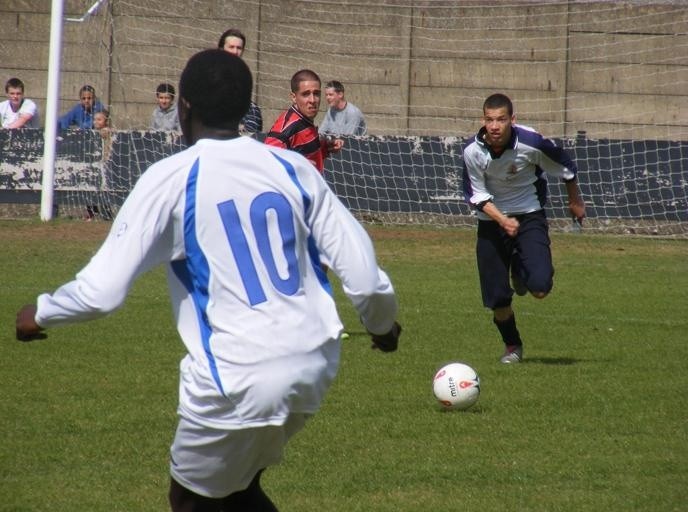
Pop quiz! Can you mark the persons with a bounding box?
[264,69,344,176]
[462,94,585,365]
[17,48,402,512]
[58,87,105,220]
[0,79,40,131]
[93,110,113,220]
[218,30,262,133]
[151,83,185,138]
[318,80,368,137]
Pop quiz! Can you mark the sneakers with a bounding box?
[500,345,523,364]
[510,252,528,296]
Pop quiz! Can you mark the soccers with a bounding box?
[432,364,481,410]
